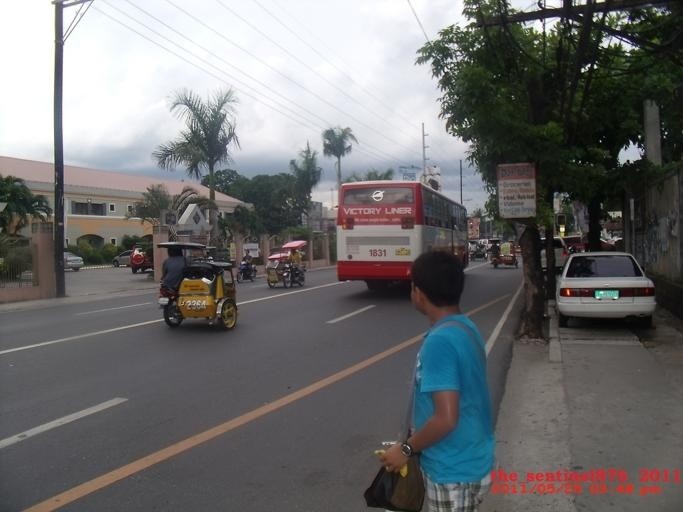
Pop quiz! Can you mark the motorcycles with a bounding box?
[236,259,257,283]
[282,261,306,290]
[488,238,499,268]
[157,271,214,328]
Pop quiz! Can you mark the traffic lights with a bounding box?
[554,215,566,229]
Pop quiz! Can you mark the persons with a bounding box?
[243,249,254,282]
[287,250,304,280]
[486,243,501,262]
[377,249,495,512]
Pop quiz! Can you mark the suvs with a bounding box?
[130,244,153,276]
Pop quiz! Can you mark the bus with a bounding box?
[336,180,469,295]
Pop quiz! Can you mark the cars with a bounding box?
[469,238,522,260]
[111,250,136,268]
[539,234,623,273]
[63,251,85,272]
[555,252,657,329]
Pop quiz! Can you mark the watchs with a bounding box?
[401,441,413,457]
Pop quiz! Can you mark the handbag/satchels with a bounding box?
[364,429,426,511]
